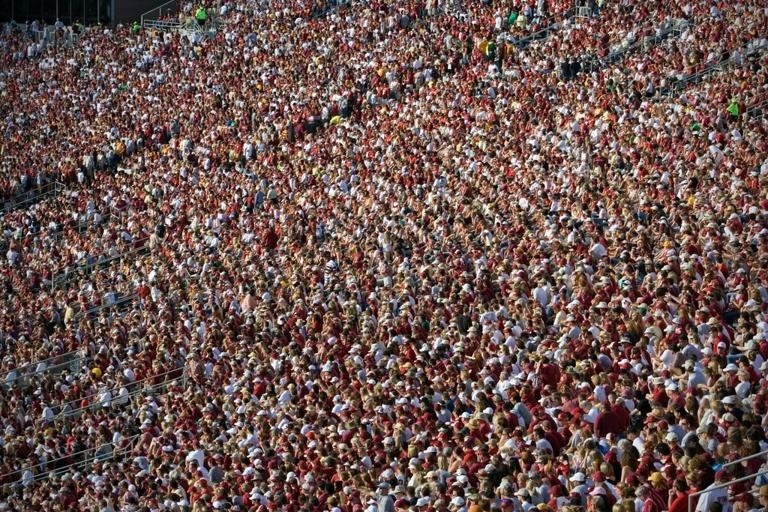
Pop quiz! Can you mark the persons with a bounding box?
[1,1,768,511]
